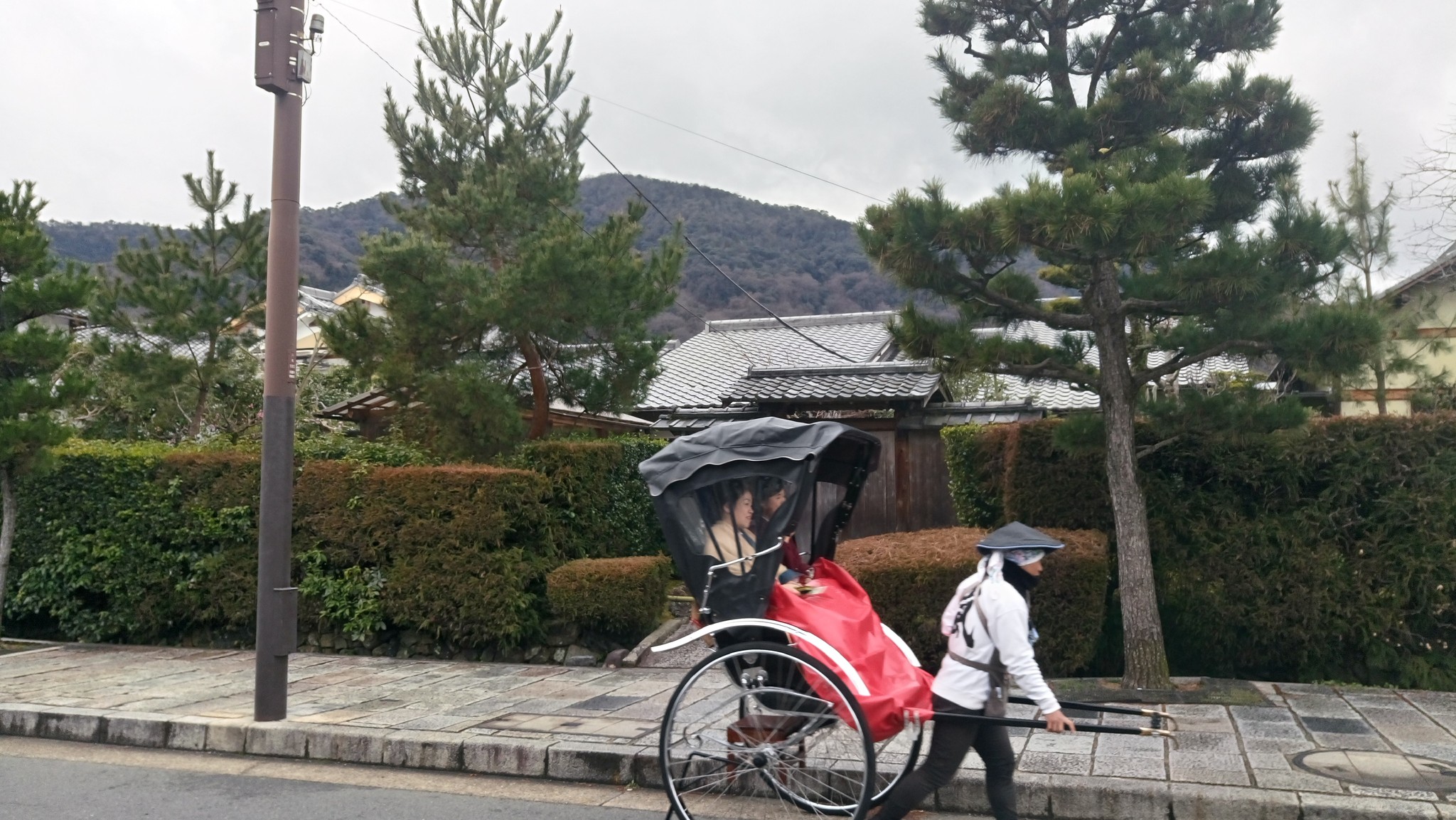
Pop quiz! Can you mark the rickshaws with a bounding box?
[636,418,1180,819]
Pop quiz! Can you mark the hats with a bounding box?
[975,521,1065,555]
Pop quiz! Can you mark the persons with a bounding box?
[748,480,816,585]
[703,484,804,597]
[866,521,1075,820]
